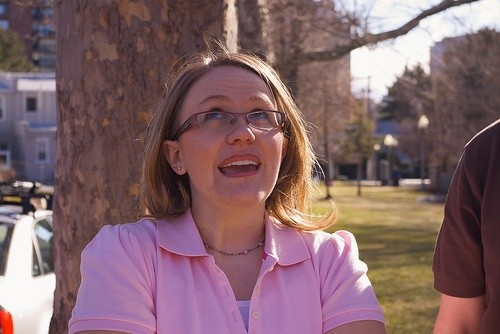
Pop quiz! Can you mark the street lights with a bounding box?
[417,114,430,182]
[384,134,395,181]
[373,144,382,179]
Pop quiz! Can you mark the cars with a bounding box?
[0,208,57,334]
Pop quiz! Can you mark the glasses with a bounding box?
[172,110,289,141]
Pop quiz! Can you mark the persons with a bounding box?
[68,53,386,334]
[432,118,500,334]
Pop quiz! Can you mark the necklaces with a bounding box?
[203,240,264,256]
